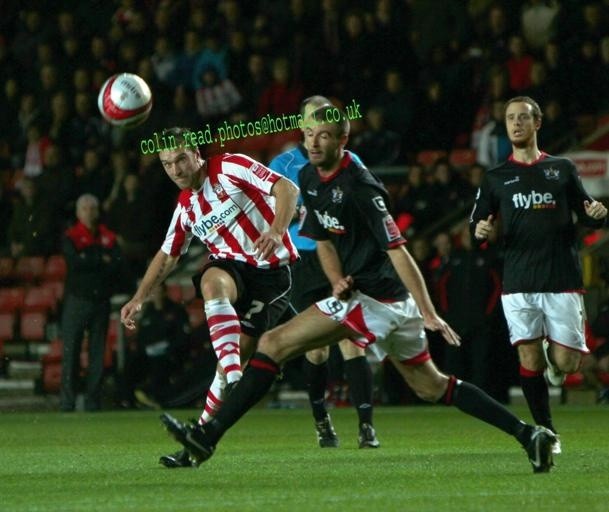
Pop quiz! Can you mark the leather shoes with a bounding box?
[83,399,99,411]
[59,400,75,410]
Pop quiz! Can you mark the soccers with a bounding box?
[97,73,152,126]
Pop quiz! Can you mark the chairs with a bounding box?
[0,252,207,394]
[415,149,477,172]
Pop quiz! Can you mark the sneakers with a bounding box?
[160,411,215,464]
[549,432,561,455]
[543,337,568,386]
[357,423,380,450]
[522,423,557,473]
[158,445,199,469]
[314,412,339,450]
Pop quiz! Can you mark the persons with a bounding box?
[1,1,608,475]
